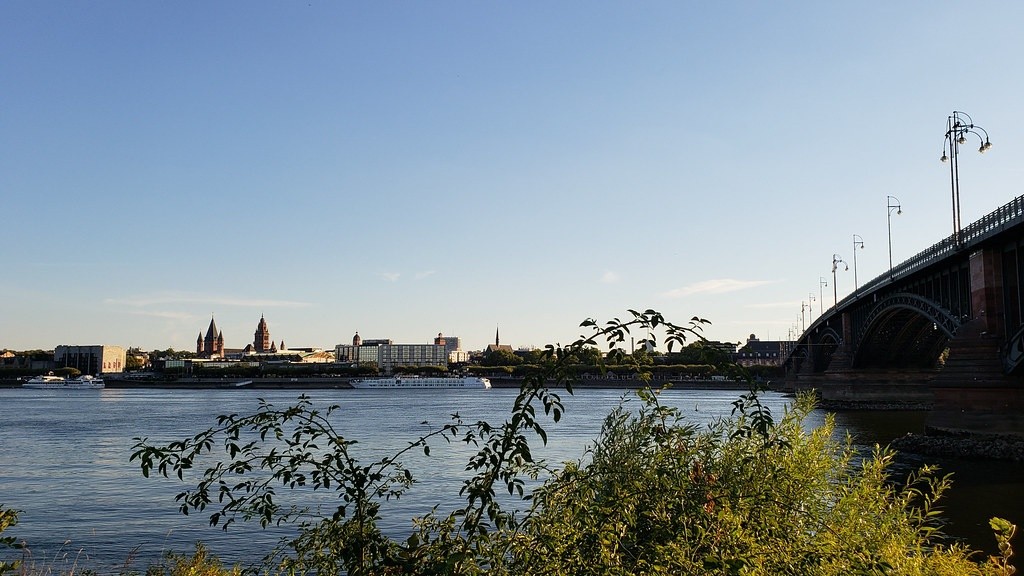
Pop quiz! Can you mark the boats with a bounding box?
[21,374,105,389]
[347,373,493,389]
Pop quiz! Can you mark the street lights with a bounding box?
[800,300,813,333]
[852,234,865,298]
[831,253,850,308]
[779,314,803,362]
[939,110,993,244]
[808,293,816,325]
[819,276,828,315]
[886,195,903,279]
